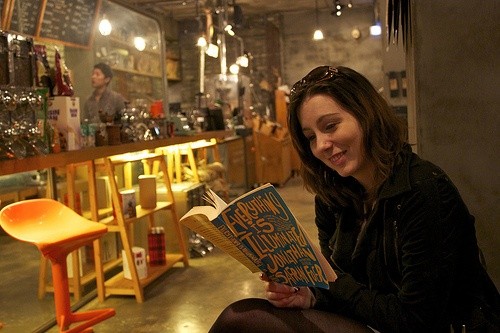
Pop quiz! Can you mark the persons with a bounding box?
[84,63,127,123]
[208,66,500,333]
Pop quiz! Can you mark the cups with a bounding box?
[105,124,121,146]
[148,227,166,267]
[151,100,164,118]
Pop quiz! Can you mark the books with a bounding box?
[178,182,337,289]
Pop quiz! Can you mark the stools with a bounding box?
[0,197,116,333]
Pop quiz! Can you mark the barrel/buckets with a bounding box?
[112,188,136,220]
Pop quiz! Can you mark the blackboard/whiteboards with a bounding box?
[1,0,9,31]
[6,1,43,40]
[37,0,103,50]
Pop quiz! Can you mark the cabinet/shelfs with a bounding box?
[37,139,240,304]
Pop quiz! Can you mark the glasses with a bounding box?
[290,66,339,98]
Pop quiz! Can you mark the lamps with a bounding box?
[313,0,324,40]
